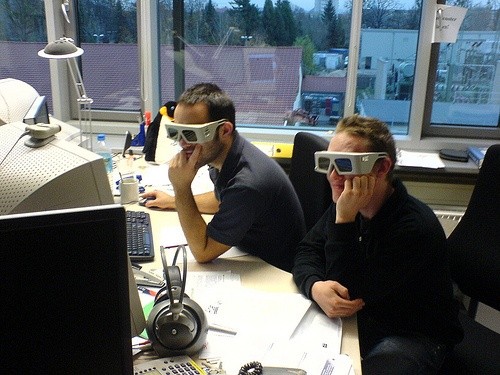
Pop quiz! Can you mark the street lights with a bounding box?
[240,35,253,45]
[93,33,104,43]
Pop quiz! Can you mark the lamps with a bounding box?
[38,37,93,153]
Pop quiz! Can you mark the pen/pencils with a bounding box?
[134,174,136,182]
[139,109,144,121]
[119,172,124,183]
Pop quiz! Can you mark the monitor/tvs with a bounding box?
[0,76,146,375]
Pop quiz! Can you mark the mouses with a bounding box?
[139,197,156,207]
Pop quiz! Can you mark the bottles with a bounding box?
[93,134,113,173]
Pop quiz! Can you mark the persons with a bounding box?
[142,83,306,275]
[293,114,464,375]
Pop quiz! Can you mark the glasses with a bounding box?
[313,151,391,175]
[164,118,229,144]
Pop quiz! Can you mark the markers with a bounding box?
[145,112,151,126]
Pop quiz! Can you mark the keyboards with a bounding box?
[125,210,154,262]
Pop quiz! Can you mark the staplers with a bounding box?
[116,175,145,192]
[131,263,165,288]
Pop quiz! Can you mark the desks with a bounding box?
[108,153,362,375]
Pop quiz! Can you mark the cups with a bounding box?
[144,112,151,125]
[120,179,139,204]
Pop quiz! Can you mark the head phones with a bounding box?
[146,245,209,357]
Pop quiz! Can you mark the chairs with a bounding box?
[444,143,500,375]
[289,132,333,232]
